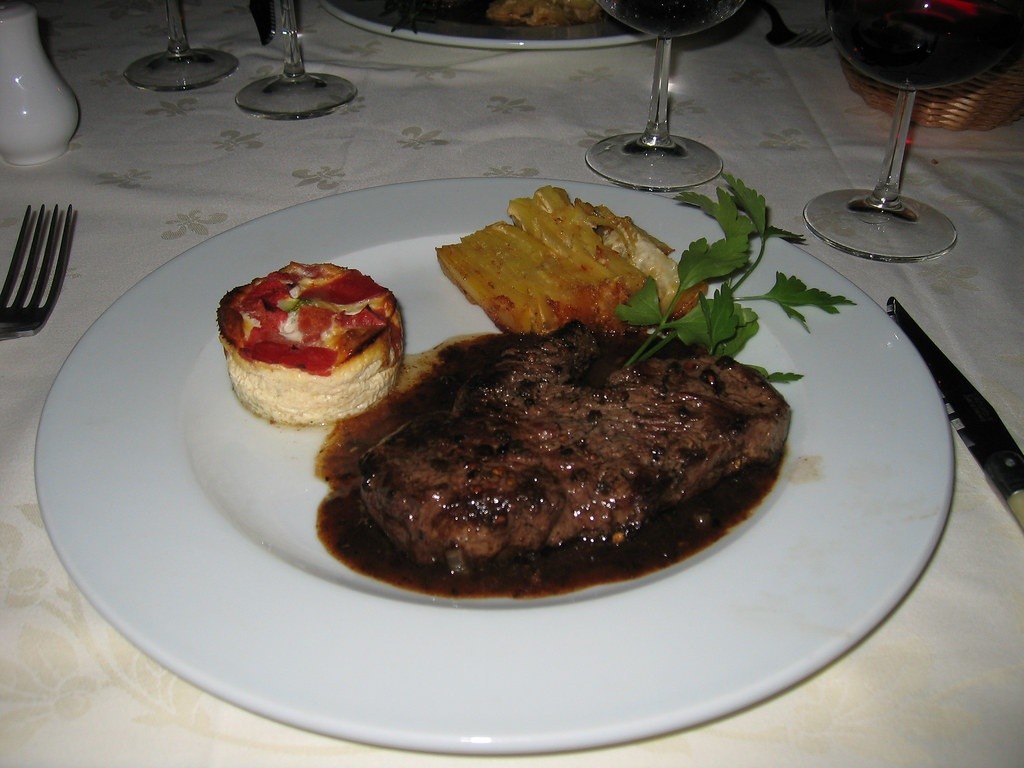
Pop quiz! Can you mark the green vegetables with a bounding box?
[613,169,857,384]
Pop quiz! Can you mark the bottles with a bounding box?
[0,1,78,166]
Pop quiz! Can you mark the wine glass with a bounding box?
[121,1,239,91]
[236,0,358,120]
[803,1,1021,264]
[585,0,745,191]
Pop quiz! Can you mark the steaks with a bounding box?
[359,321,790,566]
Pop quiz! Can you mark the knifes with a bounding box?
[887,295,1024,538]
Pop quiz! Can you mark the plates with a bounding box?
[320,0,657,50]
[35,176,954,759]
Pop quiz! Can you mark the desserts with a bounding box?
[216,262,403,426]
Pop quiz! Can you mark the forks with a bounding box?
[0,202,73,341]
[757,1,833,50]
